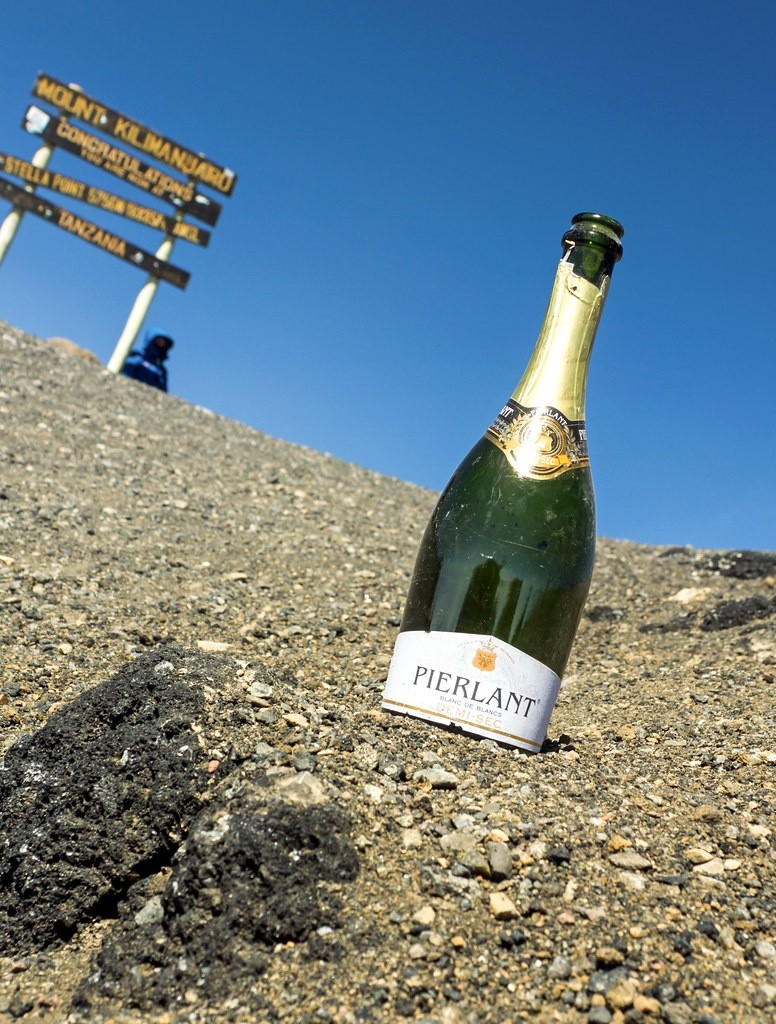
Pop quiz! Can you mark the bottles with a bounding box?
[380,212,624,755]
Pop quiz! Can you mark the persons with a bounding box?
[120,325,175,393]
